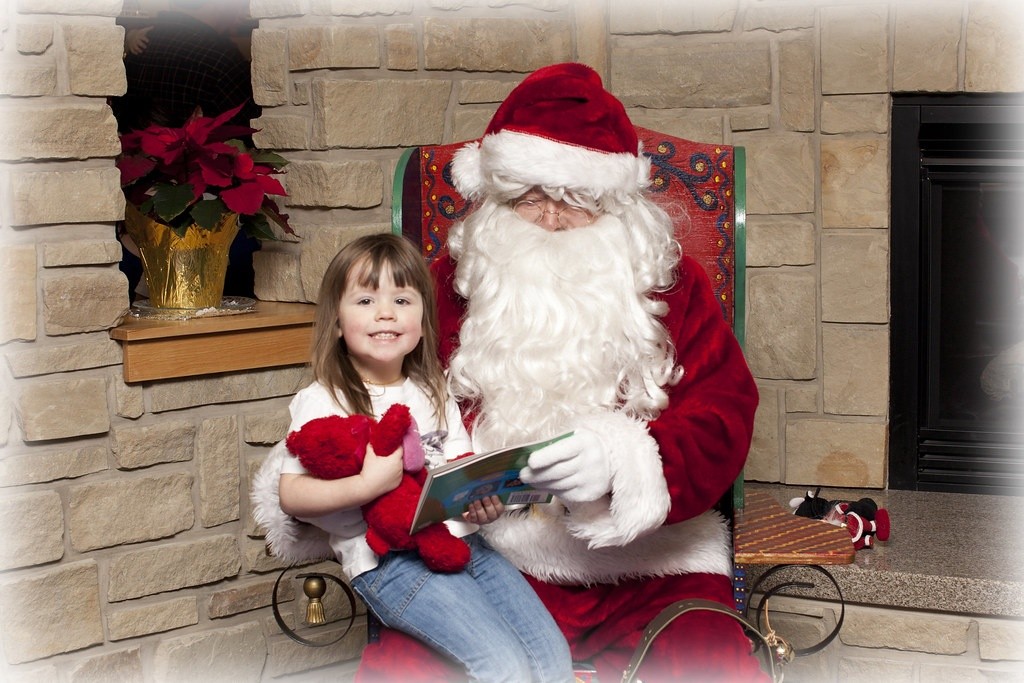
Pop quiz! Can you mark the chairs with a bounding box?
[270,122,855,683]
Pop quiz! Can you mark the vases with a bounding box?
[125,200,242,309]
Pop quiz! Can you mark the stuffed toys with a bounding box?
[287,404,472,573]
[789,487,890,549]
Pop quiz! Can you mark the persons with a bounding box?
[252,63,770,683]
[278,232,573,683]
[125,25,154,55]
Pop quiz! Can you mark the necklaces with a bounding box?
[364,375,402,385]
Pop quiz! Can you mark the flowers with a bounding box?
[115,100,305,245]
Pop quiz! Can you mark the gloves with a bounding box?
[519,433,609,502]
[296,505,368,538]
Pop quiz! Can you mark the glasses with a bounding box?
[509,200,601,230]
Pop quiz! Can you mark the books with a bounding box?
[409,431,574,536]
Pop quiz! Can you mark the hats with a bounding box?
[450,62,651,206]
